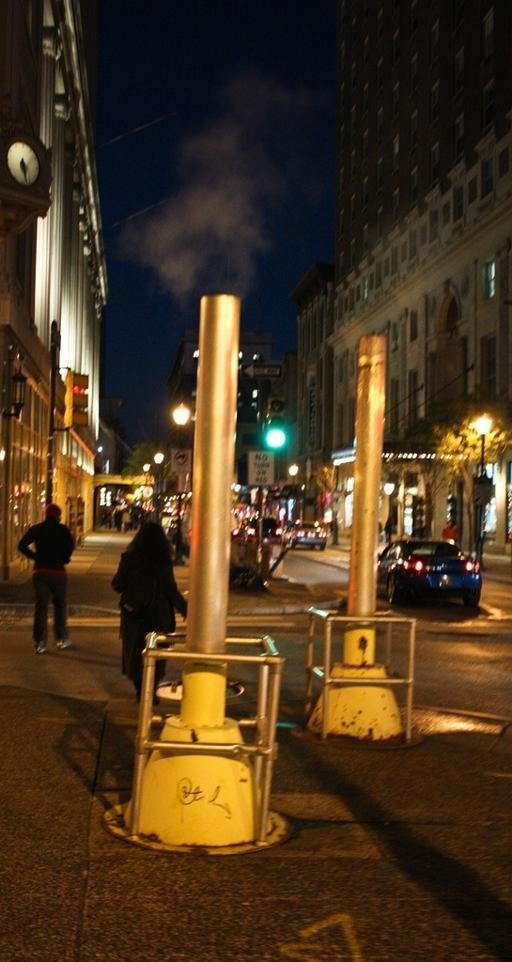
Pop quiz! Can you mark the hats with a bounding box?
[45,504,61,519]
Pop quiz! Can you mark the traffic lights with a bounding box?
[166,396,196,445]
[265,394,288,453]
[61,367,92,431]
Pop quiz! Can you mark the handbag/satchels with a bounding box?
[118,585,153,618]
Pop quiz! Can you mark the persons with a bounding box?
[111,520,189,706]
[443,518,460,544]
[16,502,78,654]
[102,497,302,544]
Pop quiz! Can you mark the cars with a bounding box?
[232,530,274,573]
[378,538,482,611]
[241,514,281,545]
[281,518,327,551]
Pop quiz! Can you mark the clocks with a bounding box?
[5,135,43,188]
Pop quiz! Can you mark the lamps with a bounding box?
[1,363,29,423]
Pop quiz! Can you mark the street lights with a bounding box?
[383,482,396,548]
[142,461,149,505]
[153,453,165,493]
[288,464,299,498]
[472,412,492,568]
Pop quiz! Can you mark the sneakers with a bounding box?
[136,692,160,705]
[57,640,73,649]
[34,641,49,654]
